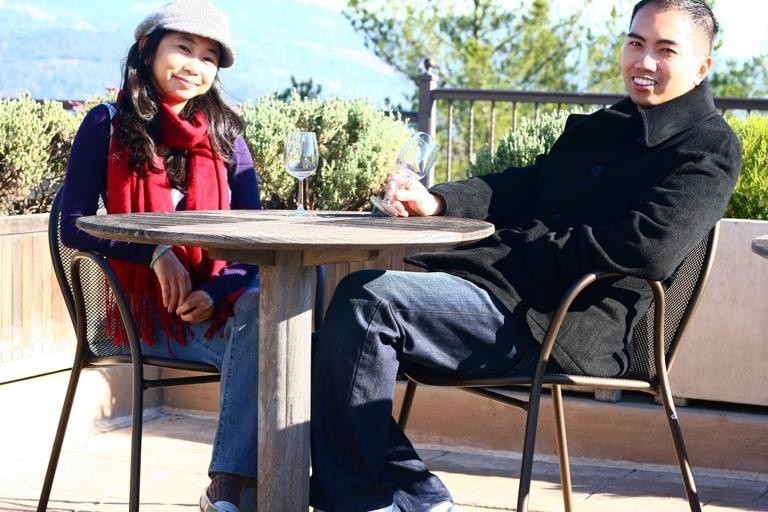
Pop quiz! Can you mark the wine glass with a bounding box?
[368,130,441,219]
[284,132,319,217]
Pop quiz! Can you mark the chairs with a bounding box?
[36,176,326,512]
[397,219,721,512]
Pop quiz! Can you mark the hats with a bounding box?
[132,0,236,70]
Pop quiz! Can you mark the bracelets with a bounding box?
[149,245,172,269]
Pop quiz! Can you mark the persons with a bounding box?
[312,1,742,512]
[59,2,327,512]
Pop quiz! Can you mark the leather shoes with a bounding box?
[198,484,228,512]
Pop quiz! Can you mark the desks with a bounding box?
[750,235,767,258]
